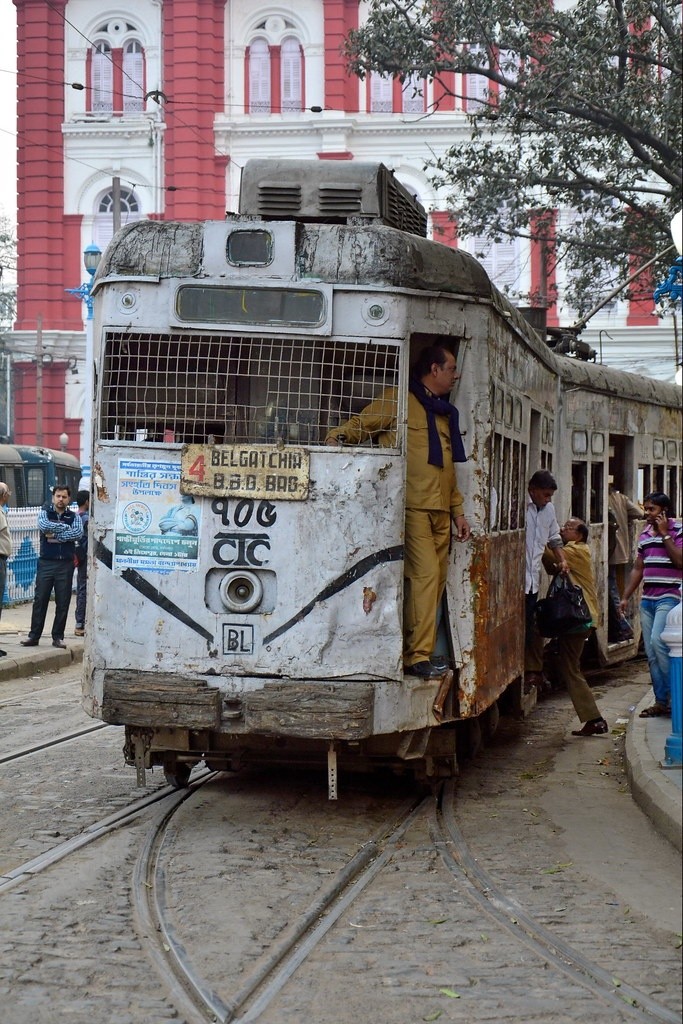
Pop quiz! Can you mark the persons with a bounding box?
[323,344,471,677]
[491,470,683,735]
[0,482,12,658]
[19,484,90,649]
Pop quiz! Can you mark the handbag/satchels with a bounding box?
[537,569,592,637]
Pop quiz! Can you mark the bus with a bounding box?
[80,154,683,789]
[0,443,83,509]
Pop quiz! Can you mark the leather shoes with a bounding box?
[407,660,450,679]
[571,718,610,737]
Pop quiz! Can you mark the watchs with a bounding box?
[662,535,671,541]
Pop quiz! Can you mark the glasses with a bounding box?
[564,525,578,531]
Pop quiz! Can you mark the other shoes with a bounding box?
[53,637,67,649]
[74,628,84,637]
[20,636,39,646]
[0,650,7,656]
[525,671,541,686]
[611,627,634,642]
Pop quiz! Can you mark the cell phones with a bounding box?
[655,507,671,524]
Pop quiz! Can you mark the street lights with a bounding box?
[63,240,103,495]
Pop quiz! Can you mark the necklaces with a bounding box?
[650,526,659,540]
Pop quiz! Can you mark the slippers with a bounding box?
[638,700,672,717]
